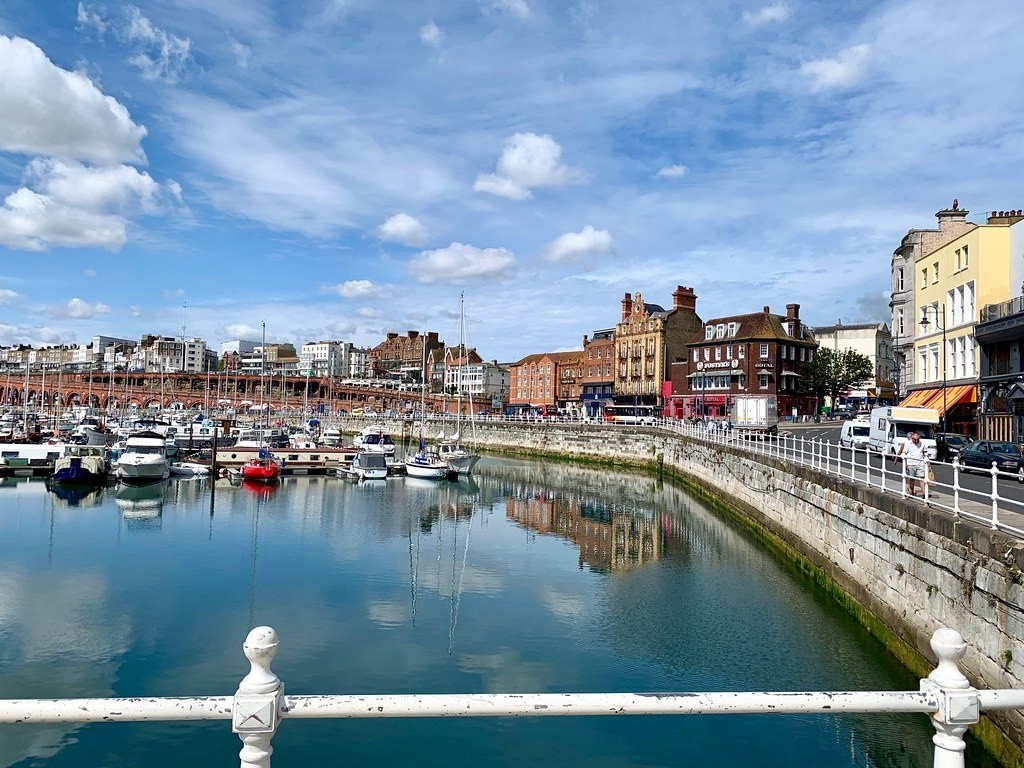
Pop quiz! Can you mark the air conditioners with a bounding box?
[984,304,1004,321]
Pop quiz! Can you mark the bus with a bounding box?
[504,403,558,424]
[603,404,659,426]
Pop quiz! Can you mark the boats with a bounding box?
[0,289,486,491]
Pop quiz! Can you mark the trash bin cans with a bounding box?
[704,417,709,427]
[812,415,820,423]
[318,404,324,413]
[794,417,798,423]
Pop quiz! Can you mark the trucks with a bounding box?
[729,392,778,441]
[868,405,941,464]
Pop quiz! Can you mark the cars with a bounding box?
[839,407,872,453]
[477,409,491,415]
[956,439,1024,483]
[936,432,976,463]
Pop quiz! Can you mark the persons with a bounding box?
[903,433,932,499]
[894,432,915,493]
[690,418,729,439]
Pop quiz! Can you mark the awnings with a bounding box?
[923,384,974,417]
[896,389,939,407]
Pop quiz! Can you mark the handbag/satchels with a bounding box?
[928,468,937,487]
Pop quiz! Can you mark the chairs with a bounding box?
[993,446,1003,452]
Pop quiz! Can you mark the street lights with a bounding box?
[918,303,946,432]
